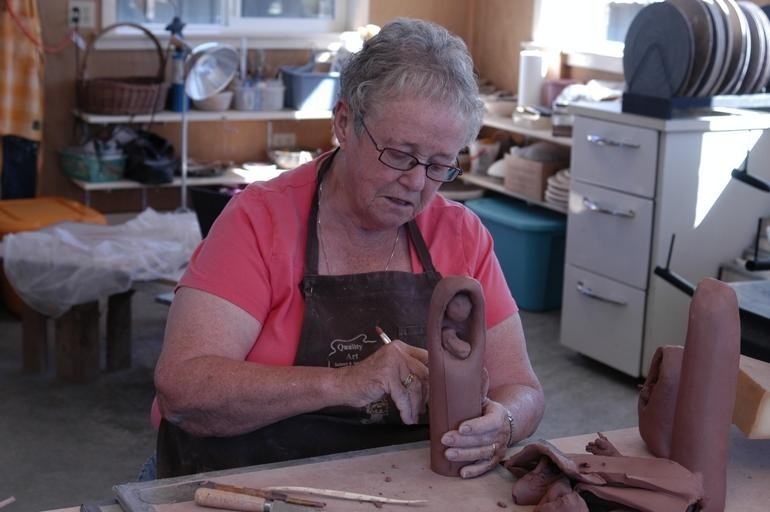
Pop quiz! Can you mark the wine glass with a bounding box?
[492,442,496,457]
[401,374,414,387]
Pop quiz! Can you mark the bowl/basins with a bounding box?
[318,177,401,276]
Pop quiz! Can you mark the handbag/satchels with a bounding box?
[508,414,514,448]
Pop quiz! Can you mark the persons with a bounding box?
[139,16,545,480]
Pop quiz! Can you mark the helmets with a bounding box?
[545,167,570,210]
[623,0,770,100]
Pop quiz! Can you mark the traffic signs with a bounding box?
[68,1,96,28]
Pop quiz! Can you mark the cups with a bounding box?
[348,110,463,184]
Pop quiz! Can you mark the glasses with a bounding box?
[156,32,240,307]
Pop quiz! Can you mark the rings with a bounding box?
[375,326,395,347]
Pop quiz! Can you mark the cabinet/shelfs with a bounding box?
[253,48,274,84]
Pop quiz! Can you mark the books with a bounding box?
[464,197,566,313]
[278,64,342,110]
[60,150,128,183]
[0,194,108,314]
[21,291,131,381]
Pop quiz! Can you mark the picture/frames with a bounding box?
[98,421,770,511]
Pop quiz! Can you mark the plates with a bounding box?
[457,115,571,214]
[69,106,187,211]
[559,101,770,379]
[187,110,485,201]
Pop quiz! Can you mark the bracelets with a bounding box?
[181,41,239,101]
[269,149,322,168]
